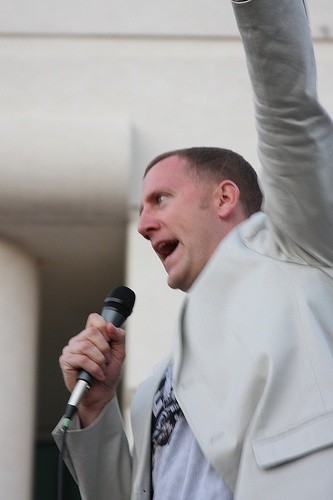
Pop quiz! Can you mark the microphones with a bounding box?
[60,285,137,432]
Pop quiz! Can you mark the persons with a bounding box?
[51,0,333,500]
